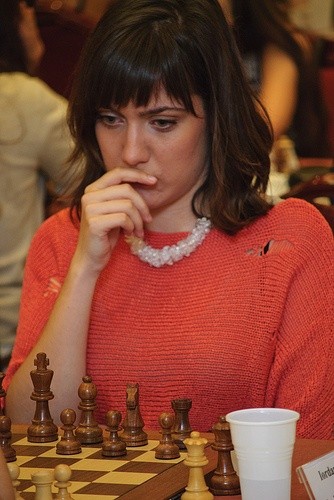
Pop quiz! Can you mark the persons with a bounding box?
[0,0,334,439]
[0,0,334,374]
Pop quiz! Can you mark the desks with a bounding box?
[208,439,334,500]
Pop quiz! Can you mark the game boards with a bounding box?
[0,423,219,500]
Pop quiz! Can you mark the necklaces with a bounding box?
[121,216,211,268]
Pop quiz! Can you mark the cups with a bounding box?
[226,408,300,500]
